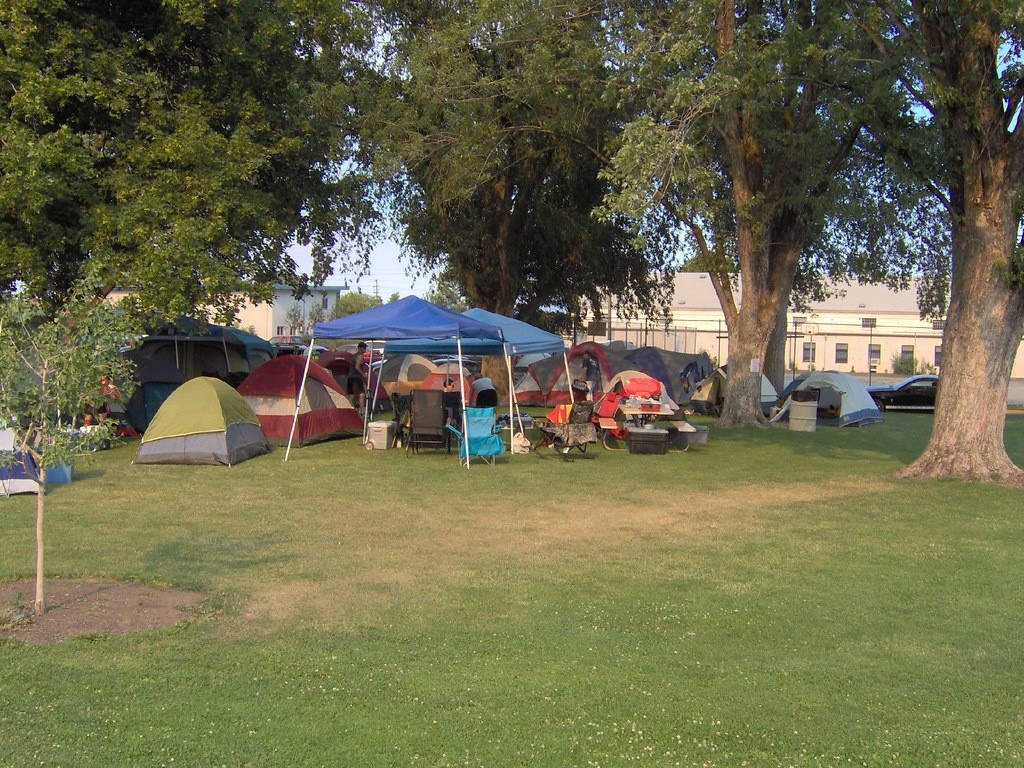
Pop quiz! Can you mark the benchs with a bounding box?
[670,420,696,453]
[599,417,622,450]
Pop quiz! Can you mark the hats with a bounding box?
[358,342,368,348]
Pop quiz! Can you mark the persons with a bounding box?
[578,350,599,402]
[81,357,112,450]
[443,378,454,392]
[347,342,369,413]
[469,372,498,408]
[303,339,329,357]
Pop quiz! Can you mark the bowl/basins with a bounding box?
[645,425,654,429]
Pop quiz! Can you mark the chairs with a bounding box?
[532,401,597,456]
[391,389,503,468]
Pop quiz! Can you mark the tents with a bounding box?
[500,338,881,427]
[369,306,576,443]
[283,294,524,469]
[134,376,270,467]
[239,355,367,447]
[0,404,72,496]
[73,310,276,434]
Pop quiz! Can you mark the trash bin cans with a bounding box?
[790,390,818,434]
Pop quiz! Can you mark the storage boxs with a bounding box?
[38,445,74,484]
[641,402,660,412]
[366,420,397,450]
[668,426,710,445]
[80,425,109,450]
[627,427,668,455]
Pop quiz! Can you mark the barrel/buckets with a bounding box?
[500,428,516,451]
[789,400,818,432]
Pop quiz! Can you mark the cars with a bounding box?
[865,375,939,413]
[268,334,554,375]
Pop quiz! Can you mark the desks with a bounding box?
[618,403,675,450]
[497,413,534,451]
